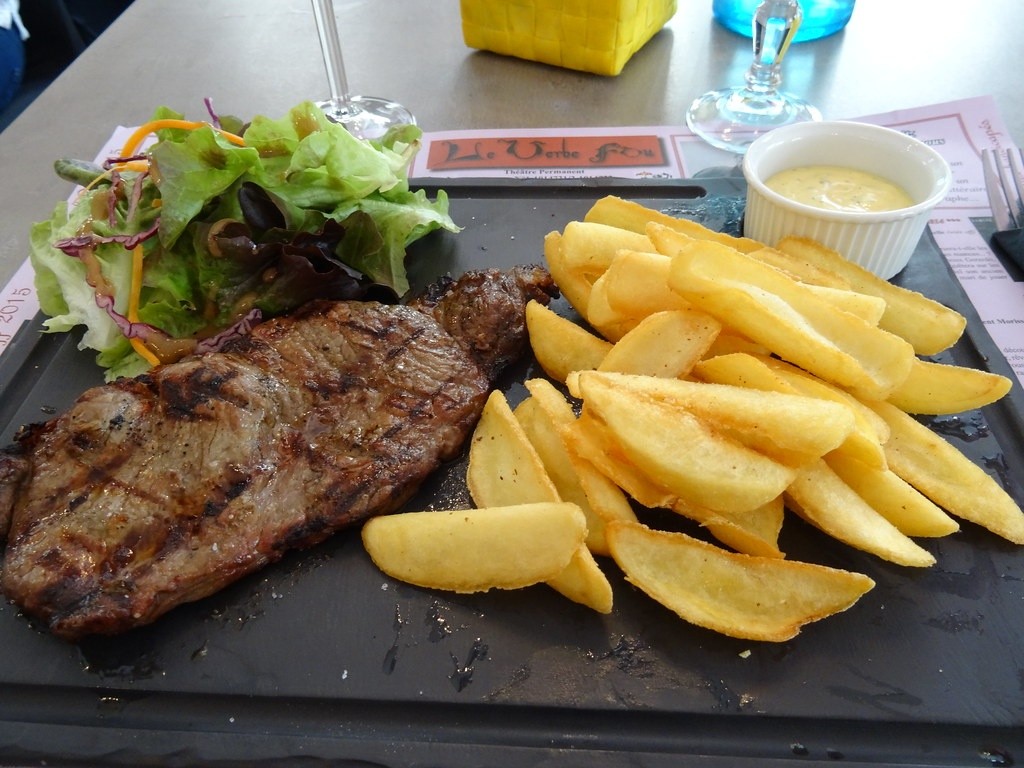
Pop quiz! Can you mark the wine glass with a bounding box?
[308,0,418,146]
[686,0,823,154]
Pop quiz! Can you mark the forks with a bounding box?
[982,149,1024,231]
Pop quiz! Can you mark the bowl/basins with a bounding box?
[740,118,953,280]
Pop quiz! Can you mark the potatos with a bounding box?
[362,192,1024,642]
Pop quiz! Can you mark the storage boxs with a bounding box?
[459,0,678,77]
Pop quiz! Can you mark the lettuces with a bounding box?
[29,101,462,381]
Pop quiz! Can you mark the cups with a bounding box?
[711,0,855,46]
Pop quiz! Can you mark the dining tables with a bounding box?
[0,0,1024,768]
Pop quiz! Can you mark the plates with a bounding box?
[0,175,1024,768]
